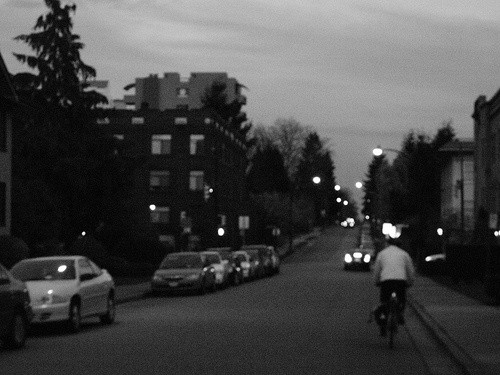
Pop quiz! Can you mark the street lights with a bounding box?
[286,172,321,256]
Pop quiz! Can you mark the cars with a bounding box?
[202,251,229,290]
[338,217,360,228]
[343,250,372,272]
[240,243,280,281]
[210,247,243,285]
[10,255,118,333]
[151,251,216,297]
[0,263,35,351]
[232,251,255,280]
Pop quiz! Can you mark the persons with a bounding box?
[372,238,416,334]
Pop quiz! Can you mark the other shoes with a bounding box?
[398,317,406,325]
[381,329,387,336]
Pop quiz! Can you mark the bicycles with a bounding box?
[374,280,412,349]
